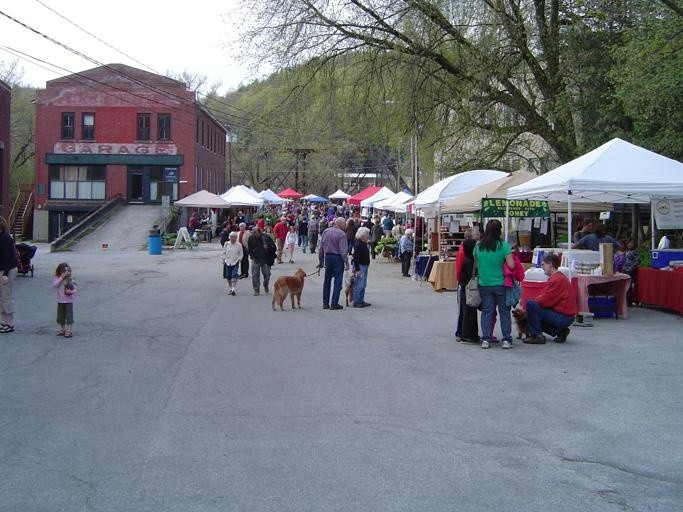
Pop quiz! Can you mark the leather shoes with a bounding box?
[237,273,248,279]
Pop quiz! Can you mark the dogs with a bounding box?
[343,277,354,307]
[271,267,307,311]
[511,308,530,340]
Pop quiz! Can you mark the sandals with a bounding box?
[56,328,73,338]
[0,322,15,334]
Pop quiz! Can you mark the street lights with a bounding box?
[383,98,419,200]
[222,123,232,190]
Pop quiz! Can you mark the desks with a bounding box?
[427,261,457,292]
[521,262,683,327]
[415,255,437,280]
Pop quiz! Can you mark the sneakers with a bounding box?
[554,328,570,343]
[330,302,344,310]
[501,340,514,350]
[481,341,491,349]
[226,288,236,295]
[522,335,546,344]
[461,336,480,344]
[323,303,329,309]
[352,302,371,308]
[253,289,260,296]
[263,282,269,293]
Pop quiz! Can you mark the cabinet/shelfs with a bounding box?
[438,210,481,260]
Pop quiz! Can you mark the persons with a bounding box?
[52,261,77,337]
[454,238,481,345]
[473,218,515,350]
[456,226,480,327]
[572,217,593,244]
[571,223,623,252]
[178,201,414,313]
[622,239,642,307]
[0,215,18,334]
[520,252,576,345]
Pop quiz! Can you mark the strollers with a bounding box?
[14,243,37,279]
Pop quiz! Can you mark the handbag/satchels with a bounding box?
[464,277,482,308]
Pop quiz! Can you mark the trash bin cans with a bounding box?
[520,267,579,318]
[147,229,161,255]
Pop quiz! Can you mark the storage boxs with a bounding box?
[532,248,600,269]
[650,249,683,269]
